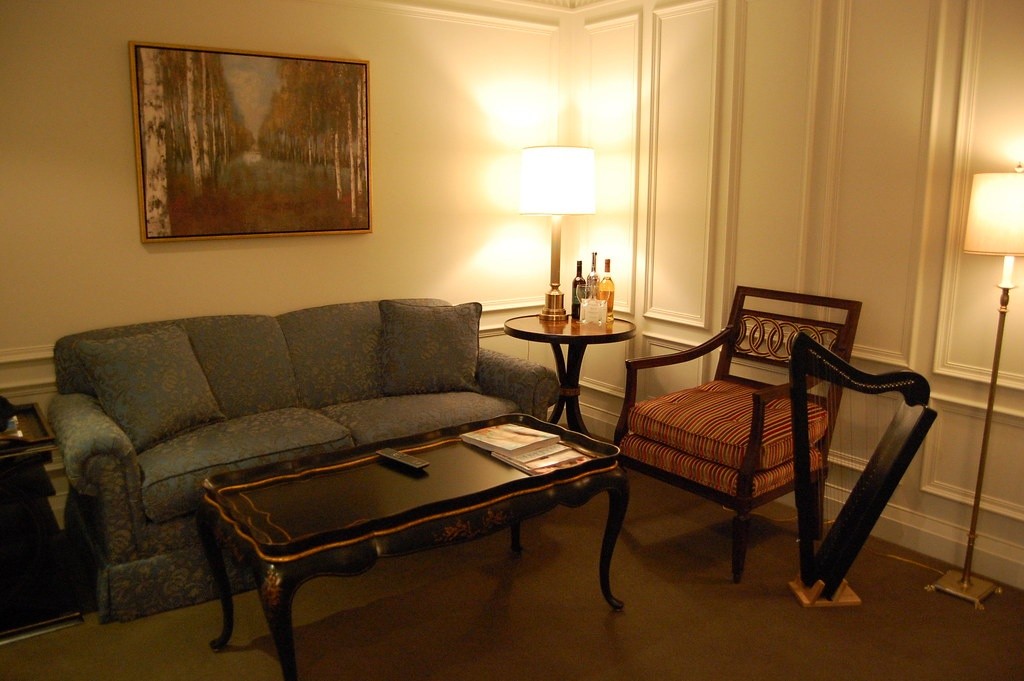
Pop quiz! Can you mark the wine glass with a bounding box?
[576,285,592,323]
[593,285,610,324]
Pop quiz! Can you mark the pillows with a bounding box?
[376,299,482,398]
[73,322,230,454]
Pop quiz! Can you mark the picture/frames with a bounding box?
[126,40,373,242]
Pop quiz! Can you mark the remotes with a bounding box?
[375,448,430,470]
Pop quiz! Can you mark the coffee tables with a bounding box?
[198,413,630,681]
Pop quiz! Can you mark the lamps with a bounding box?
[932,172,1024,602]
[517,145,598,320]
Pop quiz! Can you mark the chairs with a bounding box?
[614,285,860,584]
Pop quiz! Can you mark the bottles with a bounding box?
[600,258,615,324]
[572,262,586,321]
[587,252,600,299]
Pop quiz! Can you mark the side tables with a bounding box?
[0,401,84,643]
[504,313,637,441]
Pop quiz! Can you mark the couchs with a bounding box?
[48,297,562,627]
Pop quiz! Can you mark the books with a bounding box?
[460,424,560,455]
[491,444,593,475]
